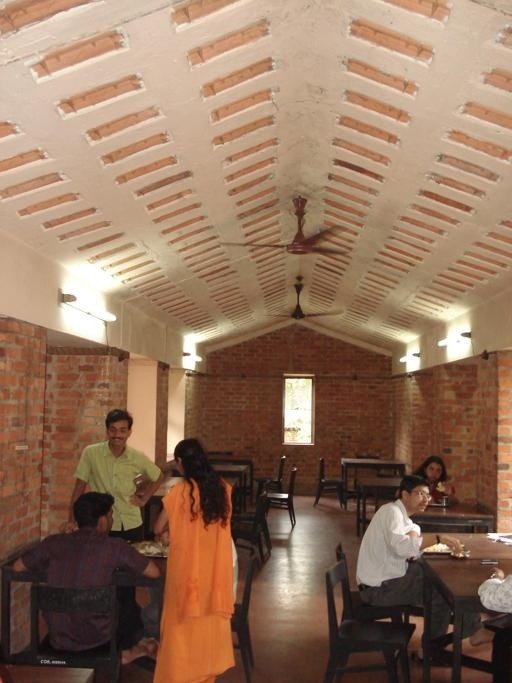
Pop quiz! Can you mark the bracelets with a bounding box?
[436,535,440,543]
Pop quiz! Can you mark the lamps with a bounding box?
[399,350,420,368]
[55,288,120,322]
[183,350,201,364]
[437,329,476,351]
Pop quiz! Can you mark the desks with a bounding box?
[139,449,273,561]
[342,457,495,534]
[412,533,510,681]
[3,533,165,682]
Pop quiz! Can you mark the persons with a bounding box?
[153,439,239,683]
[59,410,165,543]
[394,456,459,503]
[355,476,481,665]
[478,568,512,683]
[13,492,160,664]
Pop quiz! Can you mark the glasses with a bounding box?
[410,488,434,501]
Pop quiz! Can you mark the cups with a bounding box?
[439,495,448,506]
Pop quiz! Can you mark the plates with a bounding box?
[428,503,443,506]
[420,544,464,553]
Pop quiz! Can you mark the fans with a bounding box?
[259,271,351,322]
[217,192,356,261]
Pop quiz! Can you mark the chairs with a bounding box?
[228,556,260,681]
[314,457,343,507]
[324,539,419,681]
[254,452,298,530]
